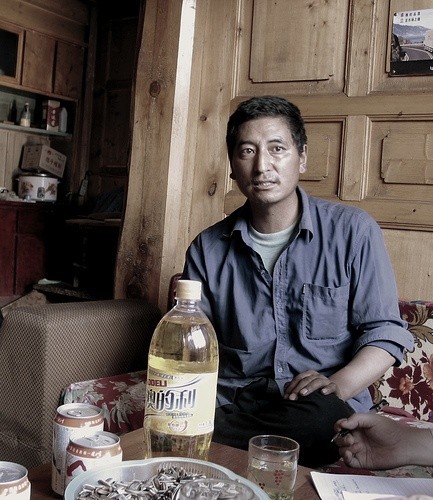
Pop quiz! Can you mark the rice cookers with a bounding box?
[15,169,61,205]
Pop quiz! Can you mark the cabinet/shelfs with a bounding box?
[0,19,85,305]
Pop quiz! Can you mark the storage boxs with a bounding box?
[19,145,67,177]
[37,100,61,132]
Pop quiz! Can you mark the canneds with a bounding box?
[51,403,104,496]
[0,461,32,500]
[64,430,122,499]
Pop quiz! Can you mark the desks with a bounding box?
[26,428,320,500]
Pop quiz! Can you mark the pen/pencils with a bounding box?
[330,399,389,443]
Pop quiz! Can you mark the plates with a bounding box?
[63,455,271,500]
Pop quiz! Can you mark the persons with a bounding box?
[333,413,433,470]
[181,95,414,471]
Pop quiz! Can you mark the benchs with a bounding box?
[0,298,433,500]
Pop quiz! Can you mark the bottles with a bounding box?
[19,102,32,127]
[59,107,67,133]
[144,279,219,461]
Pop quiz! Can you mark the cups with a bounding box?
[174,478,258,500]
[247,434,299,500]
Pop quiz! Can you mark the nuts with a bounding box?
[74,466,242,500]
